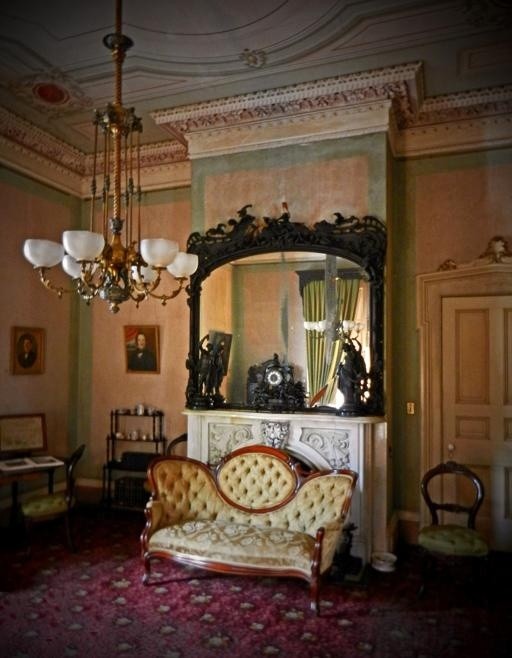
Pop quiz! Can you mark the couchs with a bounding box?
[138,445,358,615]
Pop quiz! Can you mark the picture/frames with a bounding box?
[9,325,46,375]
[121,324,160,374]
[0,413,47,459]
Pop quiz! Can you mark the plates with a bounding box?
[370,563,395,572]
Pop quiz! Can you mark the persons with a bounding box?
[194,332,215,396]
[346,332,368,398]
[18,335,36,368]
[335,332,362,404]
[127,332,156,370]
[204,339,227,397]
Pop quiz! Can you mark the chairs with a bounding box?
[20,444,86,553]
[409,461,490,606]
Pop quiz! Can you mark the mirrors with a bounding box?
[184,200,388,415]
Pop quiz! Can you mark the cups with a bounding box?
[146,407,155,415]
[129,407,136,414]
[136,404,145,416]
[130,430,140,441]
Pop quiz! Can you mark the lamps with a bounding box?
[24,0,199,314]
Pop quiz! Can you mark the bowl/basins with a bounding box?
[372,551,397,568]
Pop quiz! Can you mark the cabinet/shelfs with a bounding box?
[103,406,167,515]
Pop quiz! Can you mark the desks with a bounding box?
[0,455,68,541]
